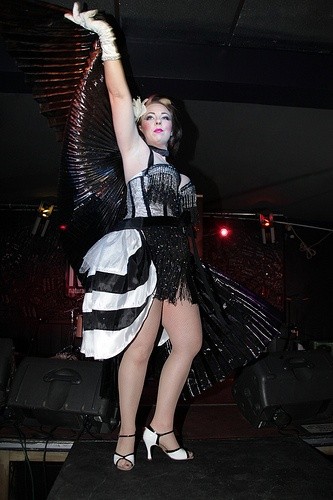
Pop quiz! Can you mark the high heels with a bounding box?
[143,424,194,461]
[113,434,135,471]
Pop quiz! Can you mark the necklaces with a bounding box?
[149,145,169,157]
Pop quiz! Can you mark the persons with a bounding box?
[64,2,202,472]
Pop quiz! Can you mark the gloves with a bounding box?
[64,2,121,61]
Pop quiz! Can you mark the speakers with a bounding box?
[0,338,120,434]
[232,349,333,428]
